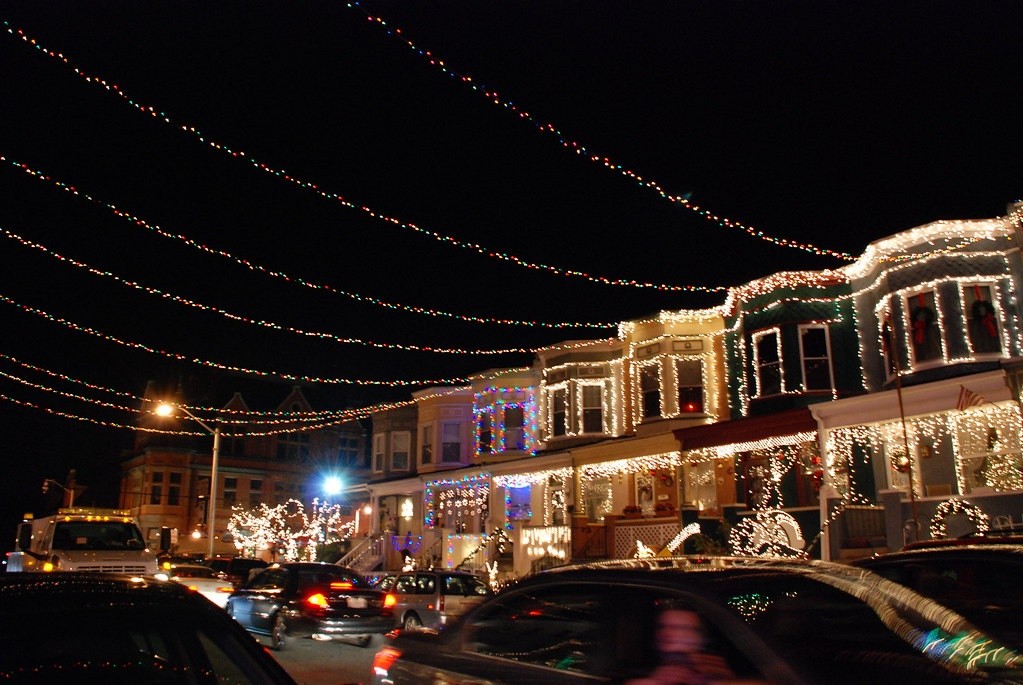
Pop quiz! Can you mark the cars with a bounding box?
[0,570,302,685]
[373,553,1023,685]
[156,552,269,611]
[371,569,497,635]
[226,560,398,649]
[834,533,1023,657]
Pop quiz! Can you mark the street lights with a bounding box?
[157,403,220,560]
[42,469,77,508]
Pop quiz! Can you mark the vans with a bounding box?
[4,504,160,580]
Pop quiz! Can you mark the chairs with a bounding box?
[992,515,1014,536]
